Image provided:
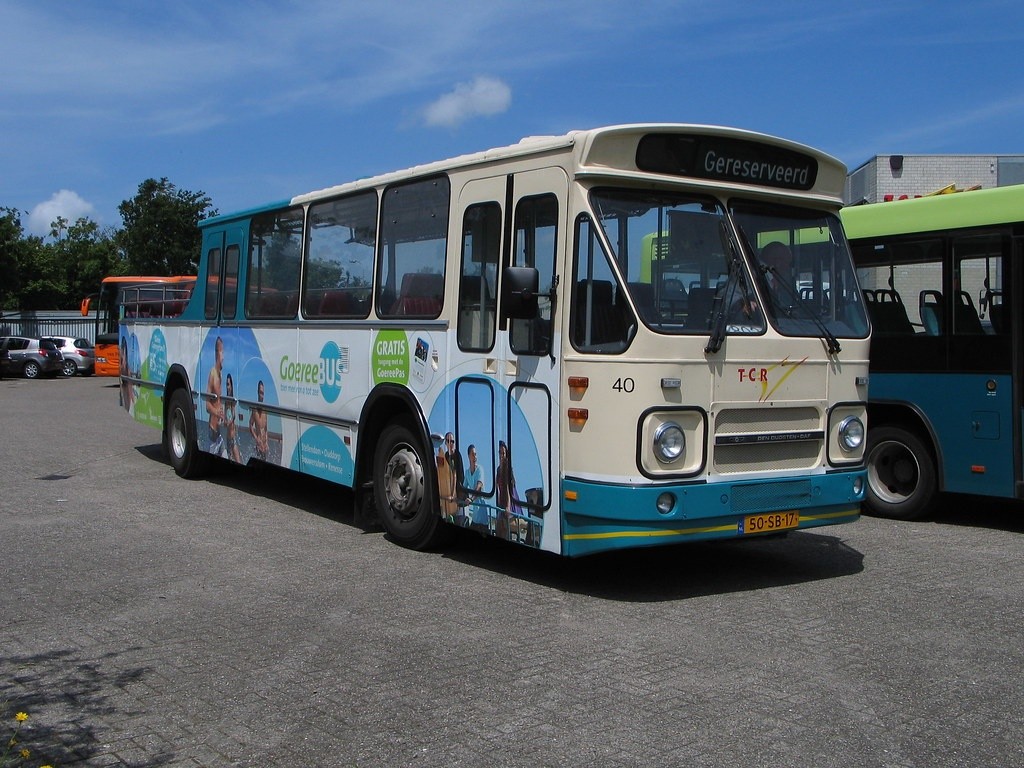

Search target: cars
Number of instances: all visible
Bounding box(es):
[0,334,94,379]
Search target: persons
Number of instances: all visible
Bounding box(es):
[205,337,269,461]
[714,241,791,323]
[442,432,523,541]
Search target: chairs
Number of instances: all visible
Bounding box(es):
[613,282,663,340]
[959,291,985,334]
[660,278,689,312]
[800,285,916,335]
[917,289,944,336]
[460,274,492,301]
[573,280,619,346]
[987,291,1004,335]
[389,271,444,317]
[317,291,354,315]
[205,294,317,316]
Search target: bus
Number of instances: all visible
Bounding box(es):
[115,118,876,565]
[640,184,1024,517]
[80,276,281,379]
